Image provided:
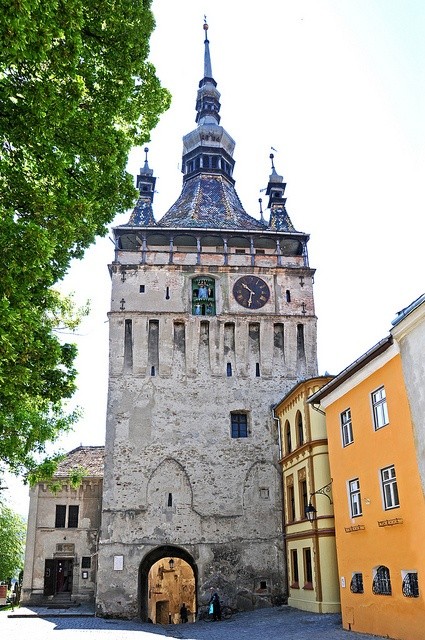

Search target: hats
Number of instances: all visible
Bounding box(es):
[214,593,217,596]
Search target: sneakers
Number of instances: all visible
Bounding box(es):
[211,619,215,622]
[218,619,222,621]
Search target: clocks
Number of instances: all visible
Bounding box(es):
[232,275,270,309]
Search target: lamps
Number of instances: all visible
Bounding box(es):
[306,478,333,523]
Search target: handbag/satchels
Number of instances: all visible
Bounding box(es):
[209,603,214,614]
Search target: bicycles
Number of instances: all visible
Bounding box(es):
[202,600,234,622]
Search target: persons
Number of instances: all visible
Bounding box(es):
[195,303,202,314]
[207,302,213,315]
[209,593,221,621]
[180,603,188,623]
